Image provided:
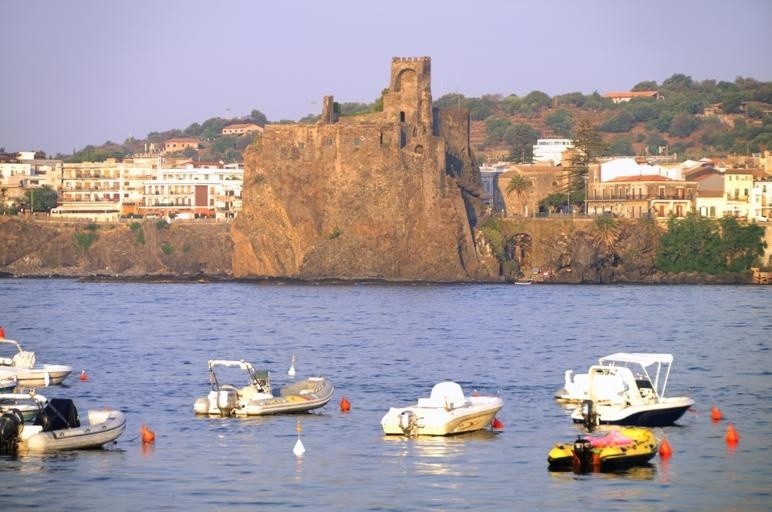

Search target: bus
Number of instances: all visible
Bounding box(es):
[45,204,120,223]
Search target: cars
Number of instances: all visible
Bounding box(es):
[602,210,616,217]
[95,272,112,279]
[566,267,573,272]
[140,212,162,219]
[754,214,768,221]
[531,267,541,275]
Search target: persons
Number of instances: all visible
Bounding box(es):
[537,200,585,216]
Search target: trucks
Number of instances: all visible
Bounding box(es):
[173,212,195,221]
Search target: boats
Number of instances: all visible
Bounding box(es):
[47,272,57,279]
[546,350,696,476]
[195,277,209,283]
[0,326,128,455]
[193,358,336,418]
[514,280,533,286]
[378,378,507,439]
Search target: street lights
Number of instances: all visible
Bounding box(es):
[162,196,169,218]
[567,190,575,214]
